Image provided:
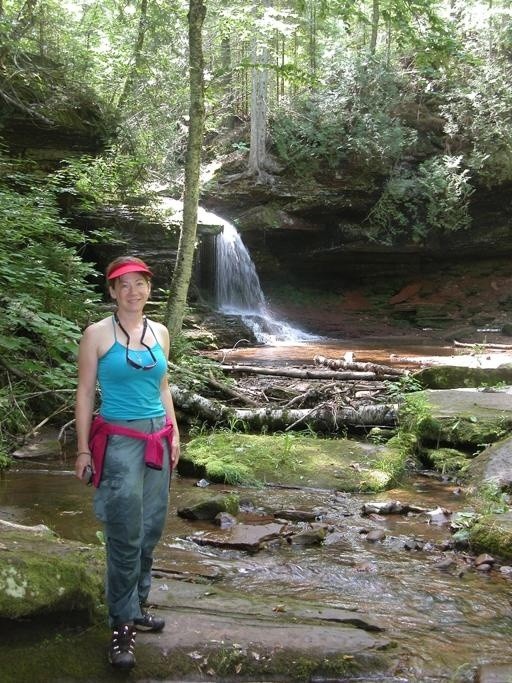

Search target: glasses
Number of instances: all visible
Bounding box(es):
[125,345,158,370]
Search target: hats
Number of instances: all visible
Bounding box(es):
[107,259,154,281]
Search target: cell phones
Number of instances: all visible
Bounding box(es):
[81,465,94,487]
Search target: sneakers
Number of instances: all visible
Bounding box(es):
[107,624,137,670]
[134,608,165,633]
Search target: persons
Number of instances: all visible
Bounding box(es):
[75,255,180,669]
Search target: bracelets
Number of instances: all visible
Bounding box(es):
[76,452,92,457]
